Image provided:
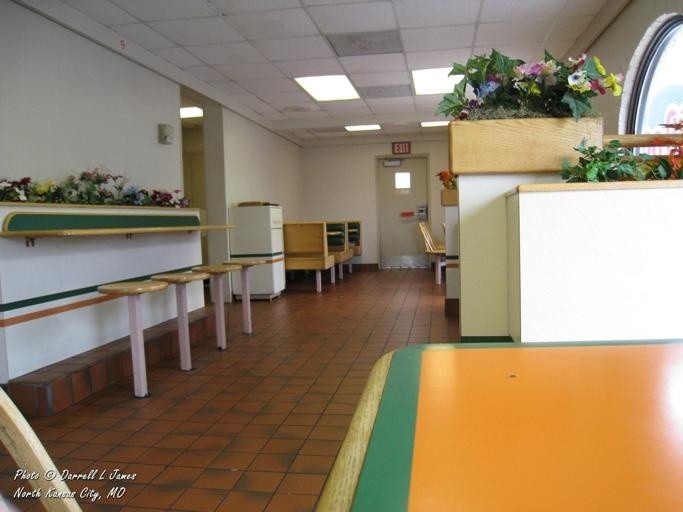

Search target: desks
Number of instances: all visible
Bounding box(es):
[311,339,682,510]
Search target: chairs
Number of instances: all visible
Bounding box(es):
[417,220,446,286]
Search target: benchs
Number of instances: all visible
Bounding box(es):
[283,219,364,292]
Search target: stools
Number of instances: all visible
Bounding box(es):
[98,260,266,399]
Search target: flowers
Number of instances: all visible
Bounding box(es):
[434,169,456,190]
[0,165,191,208]
[435,48,625,122]
